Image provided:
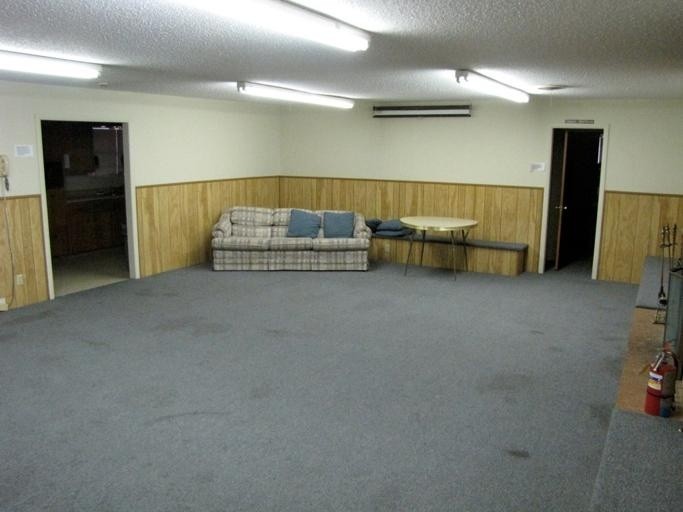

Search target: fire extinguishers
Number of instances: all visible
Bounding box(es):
[643,338,679,417]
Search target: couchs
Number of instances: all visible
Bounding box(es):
[211,206,373,270]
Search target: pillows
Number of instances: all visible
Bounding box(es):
[286,210,354,238]
[365,217,411,237]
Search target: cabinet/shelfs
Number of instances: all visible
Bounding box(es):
[67,199,125,256]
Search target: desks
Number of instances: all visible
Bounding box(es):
[398,216,477,281]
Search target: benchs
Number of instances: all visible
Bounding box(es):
[368,233,528,276]
